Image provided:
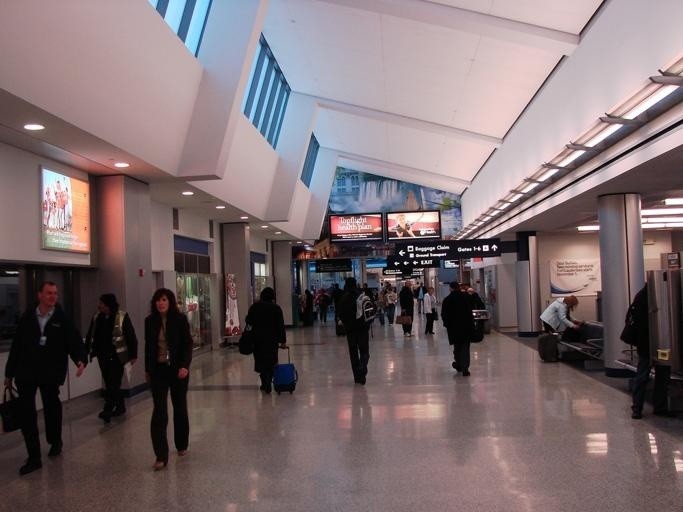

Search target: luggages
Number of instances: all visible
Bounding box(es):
[273,344,298,394]
[537,330,560,363]
[336,318,347,337]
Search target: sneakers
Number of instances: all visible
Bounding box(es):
[632,408,676,422]
[177,448,189,456]
[353,373,368,386]
[152,459,166,469]
[18,457,43,474]
[46,439,63,456]
[96,404,126,422]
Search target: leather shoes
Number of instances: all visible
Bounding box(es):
[451,361,471,376]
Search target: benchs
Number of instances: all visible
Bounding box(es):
[553,320,605,370]
[613,346,682,415]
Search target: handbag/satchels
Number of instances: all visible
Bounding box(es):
[0,381,25,433]
[237,321,257,356]
[395,311,412,325]
[620,302,639,348]
[470,321,484,344]
[432,307,439,321]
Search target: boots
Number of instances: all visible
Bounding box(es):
[260,377,272,394]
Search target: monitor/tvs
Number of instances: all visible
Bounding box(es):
[328,212,383,242]
[386,210,441,242]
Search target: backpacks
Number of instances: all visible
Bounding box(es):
[354,287,375,326]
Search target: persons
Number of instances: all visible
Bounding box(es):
[84,292,138,424]
[43,178,68,230]
[142,286,193,473]
[294,279,487,338]
[244,286,286,395]
[3,280,88,478]
[440,281,484,376]
[336,277,370,386]
[390,212,415,238]
[619,283,679,419]
[537,294,580,363]
